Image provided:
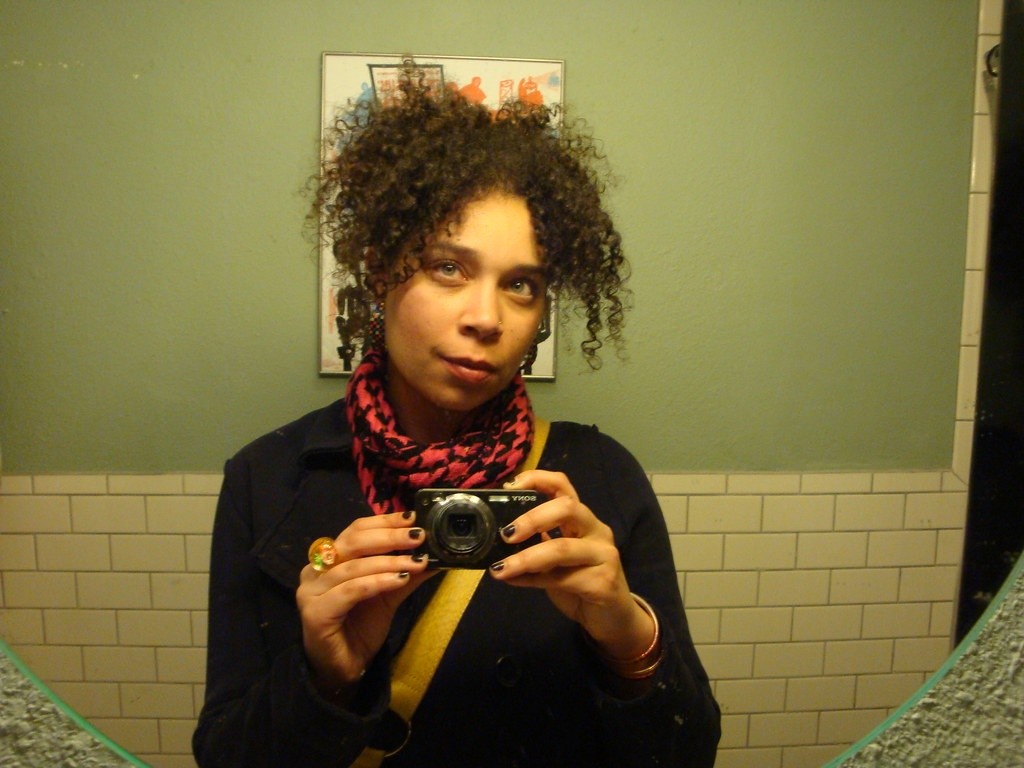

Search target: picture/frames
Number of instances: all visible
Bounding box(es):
[314,52,568,382]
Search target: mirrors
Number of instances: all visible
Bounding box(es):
[0,1,1023,767]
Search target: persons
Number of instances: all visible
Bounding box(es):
[192,76,723,768]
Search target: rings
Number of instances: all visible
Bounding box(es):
[308,538,339,571]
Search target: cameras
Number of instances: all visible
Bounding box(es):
[413,489,542,571]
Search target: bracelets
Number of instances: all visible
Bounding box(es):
[621,652,664,680]
[608,594,660,666]
